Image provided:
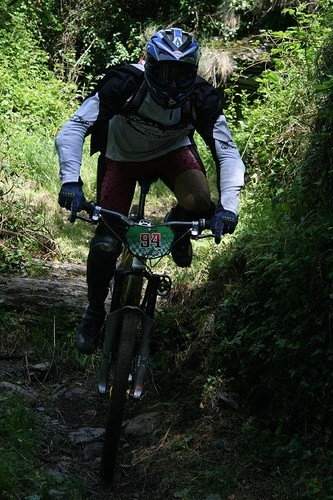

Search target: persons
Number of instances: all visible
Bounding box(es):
[54,28,245,353]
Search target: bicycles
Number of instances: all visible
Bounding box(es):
[64,176,215,487]
